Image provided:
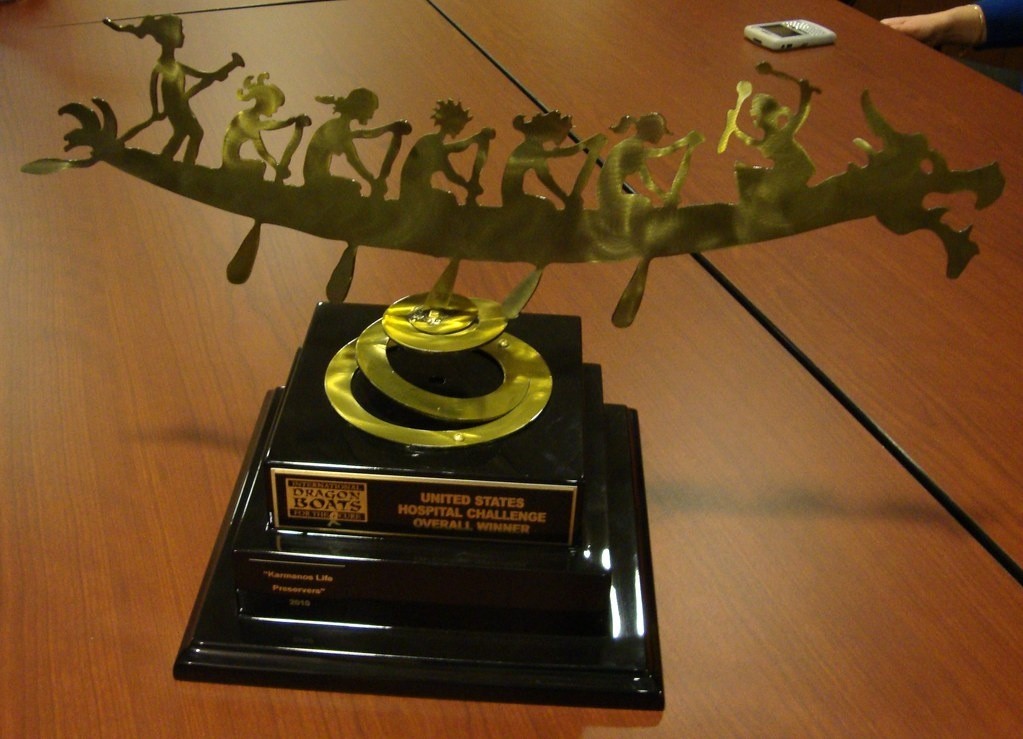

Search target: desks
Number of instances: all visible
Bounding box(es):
[1,1,1023,739]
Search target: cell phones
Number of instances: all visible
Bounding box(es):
[744,19,837,51]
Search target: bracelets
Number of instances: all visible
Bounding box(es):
[966,4,984,42]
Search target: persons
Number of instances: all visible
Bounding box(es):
[102,11,816,219]
[879,0,1023,52]
